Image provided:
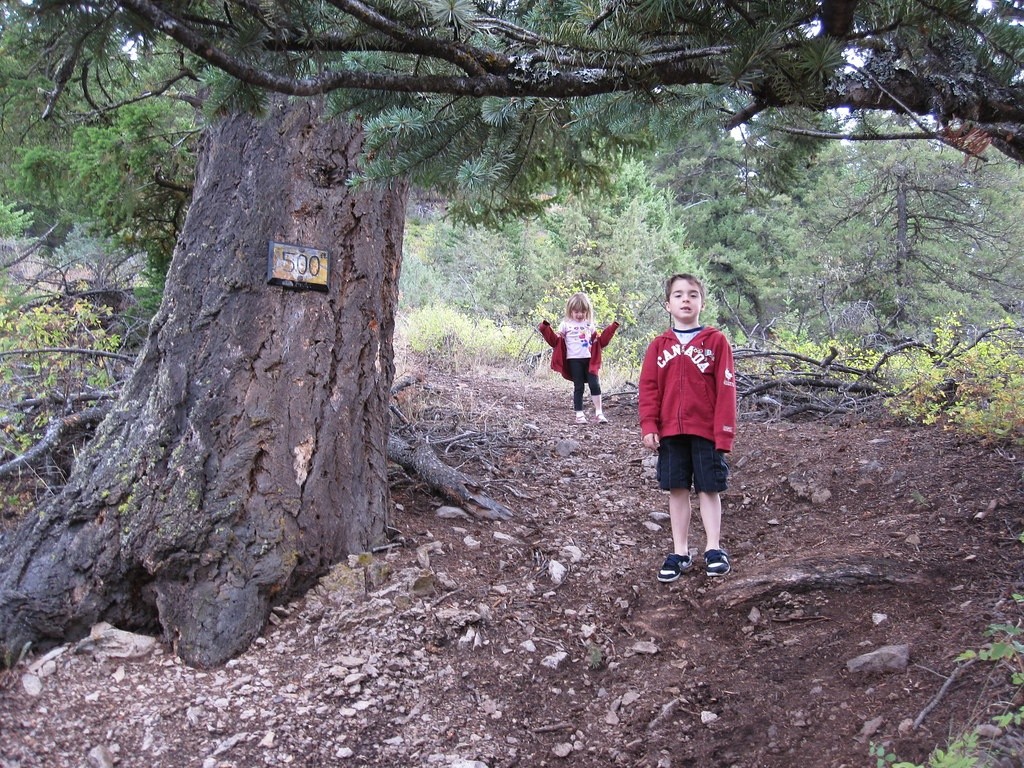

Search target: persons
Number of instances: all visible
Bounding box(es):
[537,292,620,424]
[638,274,736,582]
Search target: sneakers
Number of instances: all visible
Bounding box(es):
[704,548,731,577]
[576,417,587,423]
[657,550,693,583]
[596,414,608,423]
